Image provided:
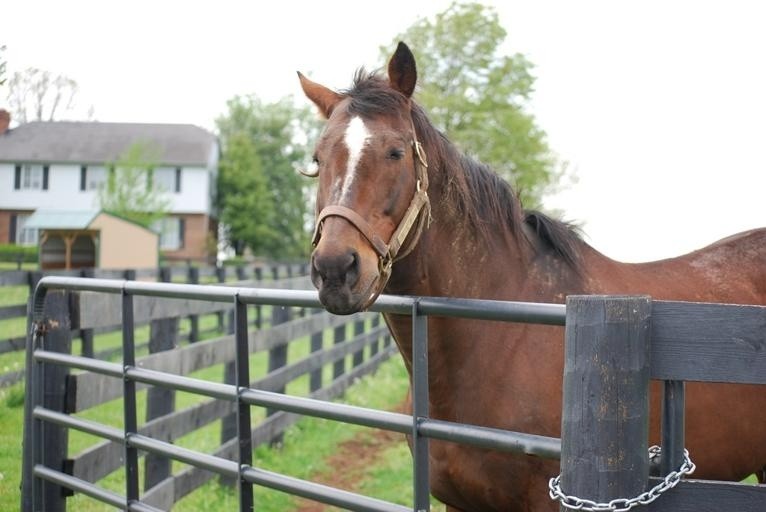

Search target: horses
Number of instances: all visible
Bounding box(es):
[296,40,766,512]
